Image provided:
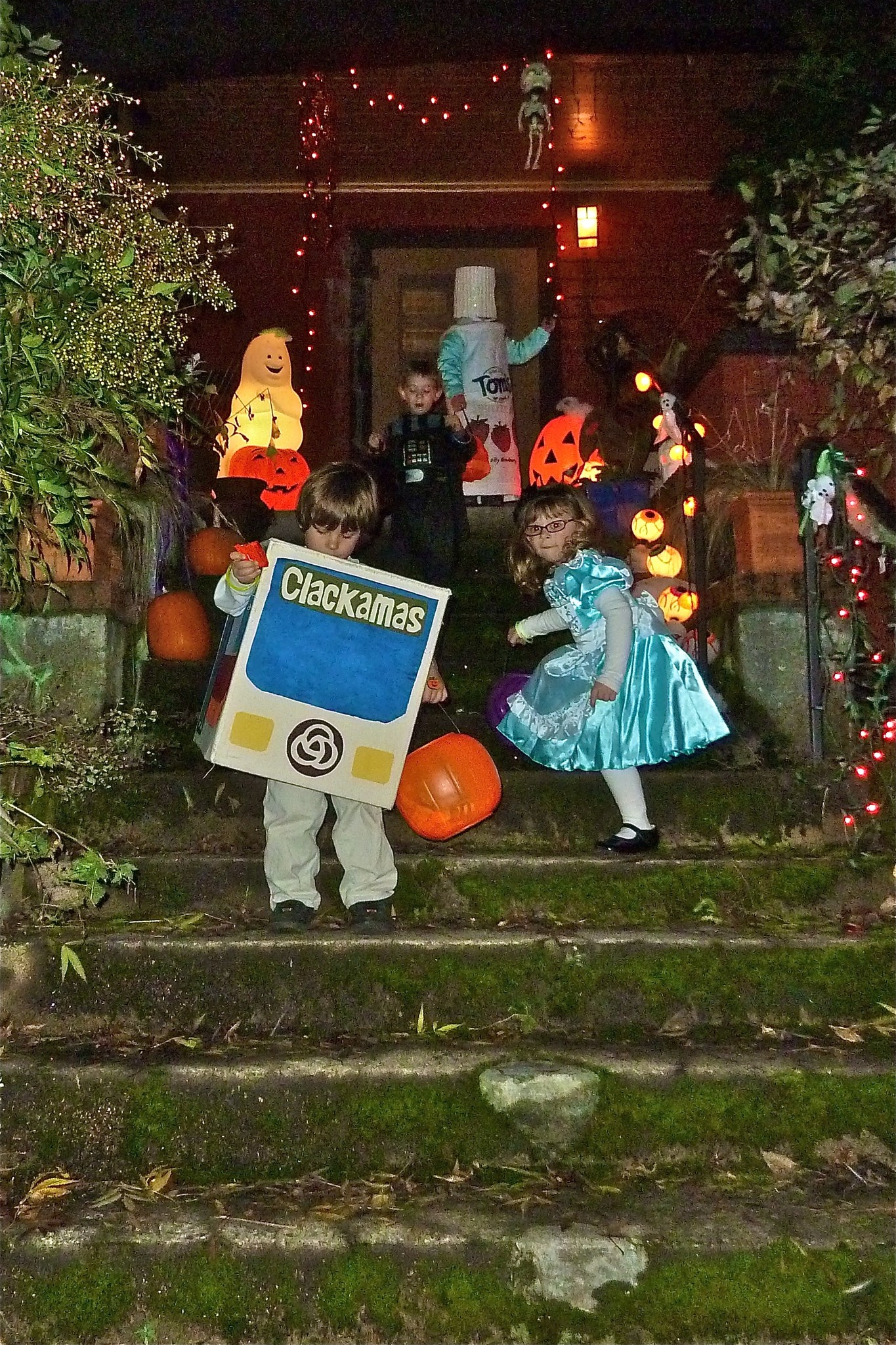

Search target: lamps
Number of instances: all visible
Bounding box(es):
[574,205,600,249]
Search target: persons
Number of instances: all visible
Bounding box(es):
[436,266,554,506]
[365,359,477,655]
[214,463,447,936]
[495,484,730,855]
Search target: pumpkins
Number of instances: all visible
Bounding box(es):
[147,585,211,660]
[189,506,245,576]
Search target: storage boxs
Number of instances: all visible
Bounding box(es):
[192,535,452,812]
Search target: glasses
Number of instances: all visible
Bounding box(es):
[523,517,574,537]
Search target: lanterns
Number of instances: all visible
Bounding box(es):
[228,446,311,511]
[529,415,604,488]
[397,732,503,841]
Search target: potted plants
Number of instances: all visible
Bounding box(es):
[683,363,822,574]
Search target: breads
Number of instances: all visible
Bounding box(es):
[235,541,269,567]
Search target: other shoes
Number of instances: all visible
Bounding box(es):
[594,822,660,853]
[348,899,393,935]
[268,900,317,931]
